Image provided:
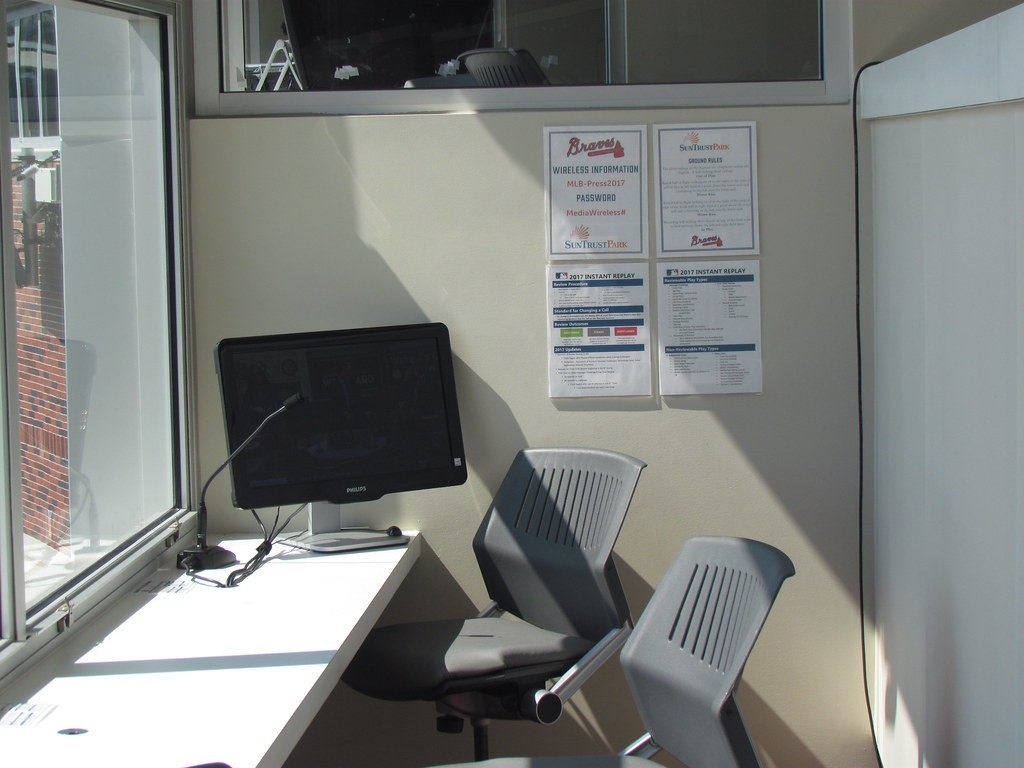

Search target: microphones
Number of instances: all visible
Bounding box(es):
[176,393,306,571]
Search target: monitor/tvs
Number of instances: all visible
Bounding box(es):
[213,322,468,553]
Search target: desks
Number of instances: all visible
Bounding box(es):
[0,531,421,768]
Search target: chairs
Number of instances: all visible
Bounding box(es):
[421,535,797,768]
[340,447,648,761]
